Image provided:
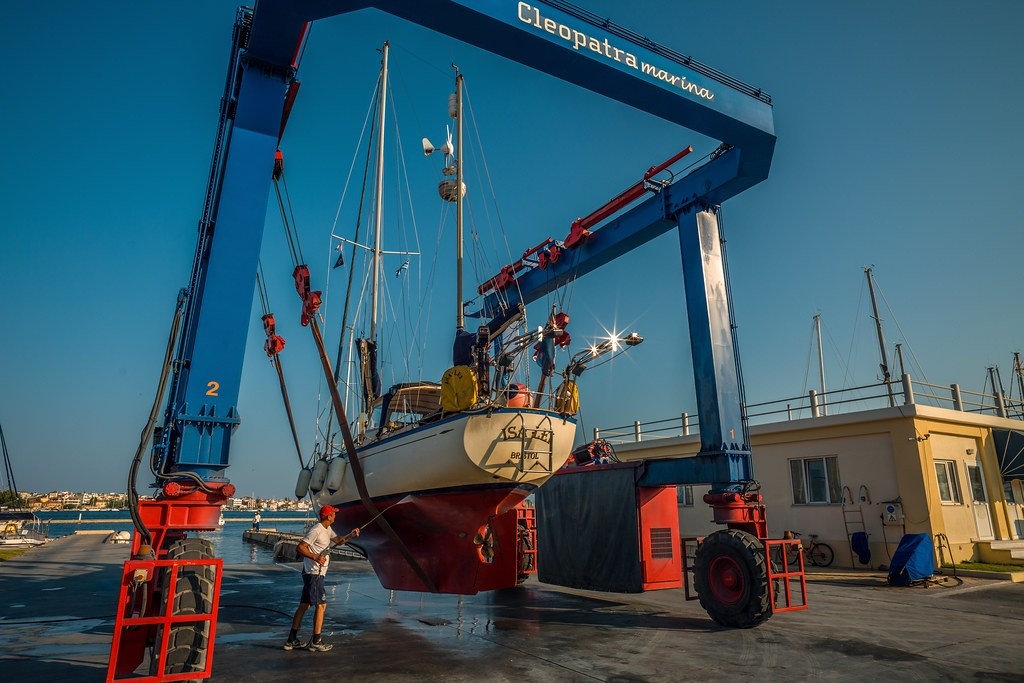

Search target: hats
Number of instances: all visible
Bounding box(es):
[319,506,339,518]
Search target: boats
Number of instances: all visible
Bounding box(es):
[0,528,48,550]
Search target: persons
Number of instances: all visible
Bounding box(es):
[256,511,261,532]
[283,506,360,651]
[253,513,257,533]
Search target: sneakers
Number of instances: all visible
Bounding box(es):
[308,637,333,652]
[283,639,307,650]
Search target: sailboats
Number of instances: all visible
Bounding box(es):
[290,42,647,507]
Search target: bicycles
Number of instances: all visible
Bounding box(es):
[778,531,834,567]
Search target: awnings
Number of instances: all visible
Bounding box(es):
[992,429,1024,482]
